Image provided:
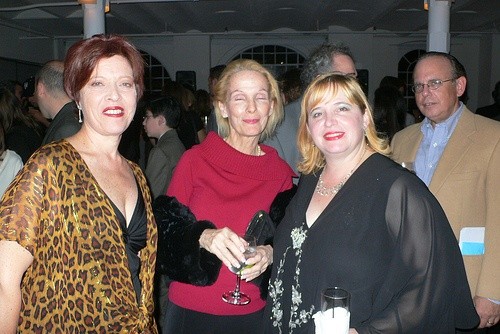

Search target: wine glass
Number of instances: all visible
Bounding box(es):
[221,234,257,305]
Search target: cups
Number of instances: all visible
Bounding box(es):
[320,287,351,318]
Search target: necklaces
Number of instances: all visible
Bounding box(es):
[316,145,368,196]
[223,136,261,156]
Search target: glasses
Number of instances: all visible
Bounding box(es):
[143,114,159,121]
[412,76,462,94]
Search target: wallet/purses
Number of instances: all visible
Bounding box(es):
[241,210,277,289]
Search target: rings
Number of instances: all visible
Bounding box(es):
[487,320,494,324]
[259,270,261,274]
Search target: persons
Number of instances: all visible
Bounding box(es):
[0,34,500,334]
